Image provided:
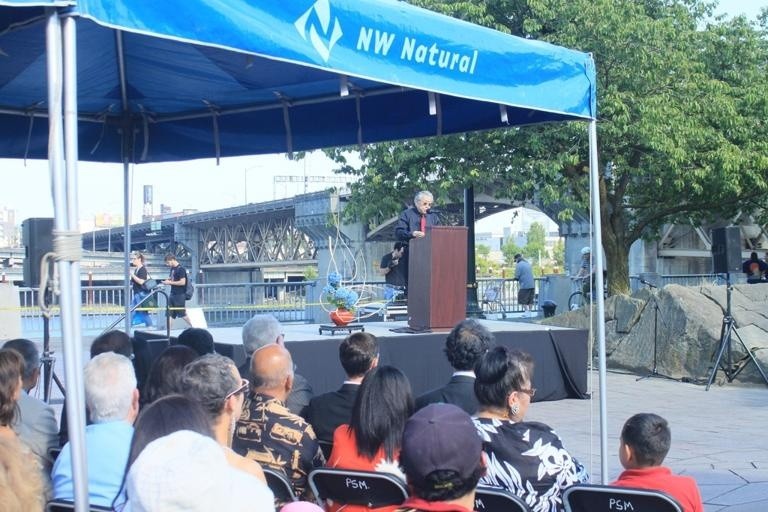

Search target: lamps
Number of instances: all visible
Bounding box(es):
[563,484,684,512]
[474,486,531,512]
[45,499,111,512]
[263,467,297,504]
[308,467,412,508]
[47,446,62,464]
[477,280,510,319]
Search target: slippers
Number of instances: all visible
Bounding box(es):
[176,266,194,300]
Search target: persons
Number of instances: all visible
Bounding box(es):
[394,403,487,512]
[0,349,42,512]
[742,251,767,283]
[412,318,493,414]
[160,254,193,328]
[395,191,442,238]
[130,253,157,327]
[308,332,380,458]
[379,241,409,300]
[174,325,216,358]
[606,412,704,512]
[51,351,141,510]
[326,365,413,512]
[182,354,271,488]
[468,345,589,512]
[574,247,597,295]
[513,254,535,318]
[227,343,326,500]
[57,331,137,450]
[237,313,314,415]
[2,338,57,471]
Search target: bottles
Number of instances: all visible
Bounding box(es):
[513,253,522,263]
[130,251,141,260]
[401,402,482,490]
[581,246,592,254]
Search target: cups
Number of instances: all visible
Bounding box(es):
[132,257,138,261]
[224,378,250,399]
[517,386,535,397]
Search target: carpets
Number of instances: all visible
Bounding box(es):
[420,214,427,232]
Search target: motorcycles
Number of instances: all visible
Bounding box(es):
[634,306,666,381]
[704,274,768,391]
[28,287,66,406]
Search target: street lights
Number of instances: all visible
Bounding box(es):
[143,268,158,291]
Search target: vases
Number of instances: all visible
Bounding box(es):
[323,271,359,310]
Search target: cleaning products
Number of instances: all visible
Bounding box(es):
[478,278,508,319]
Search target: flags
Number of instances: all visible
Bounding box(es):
[329,307,356,326]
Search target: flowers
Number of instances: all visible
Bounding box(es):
[640,279,656,288]
[426,209,441,214]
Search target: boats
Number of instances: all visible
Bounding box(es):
[712,226,743,274]
[21,217,55,287]
